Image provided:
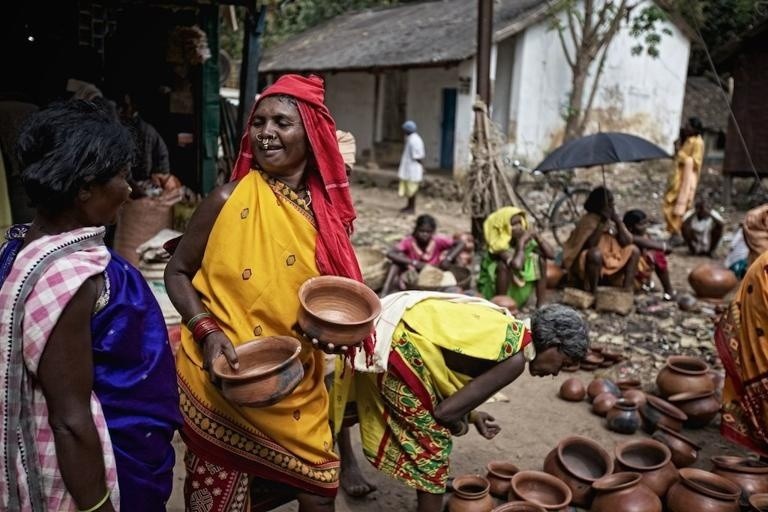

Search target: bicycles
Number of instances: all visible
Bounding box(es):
[501,156,594,247]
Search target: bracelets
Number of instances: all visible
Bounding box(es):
[187,313,222,342]
[411,260,418,267]
[86,488,113,512]
[462,412,471,422]
[663,241,667,251]
[446,256,453,262]
[540,240,545,245]
[455,421,468,437]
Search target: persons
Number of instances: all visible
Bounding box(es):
[120,104,170,181]
[336,128,376,496]
[381,214,467,298]
[353,290,591,511]
[720,248,768,457]
[164,76,367,509]
[681,192,726,259]
[665,116,706,243]
[398,121,427,213]
[486,208,555,311]
[0,100,183,512]
[624,210,679,304]
[563,187,639,293]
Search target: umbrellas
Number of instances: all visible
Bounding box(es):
[530,122,674,234]
